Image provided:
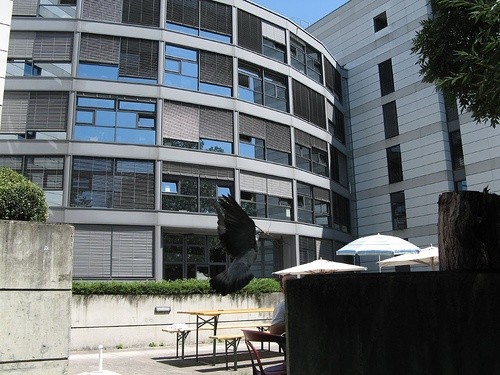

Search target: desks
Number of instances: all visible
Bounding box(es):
[177,309,274,366]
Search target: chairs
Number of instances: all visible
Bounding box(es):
[243,329,289,375]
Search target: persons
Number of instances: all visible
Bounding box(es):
[270,274,297,335]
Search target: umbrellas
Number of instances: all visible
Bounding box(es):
[376,244,440,271]
[336,233,421,272]
[273,257,367,279]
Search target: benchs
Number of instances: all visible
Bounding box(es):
[210,333,242,371]
[163,322,271,360]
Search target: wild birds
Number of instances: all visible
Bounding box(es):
[209,193,289,296]
